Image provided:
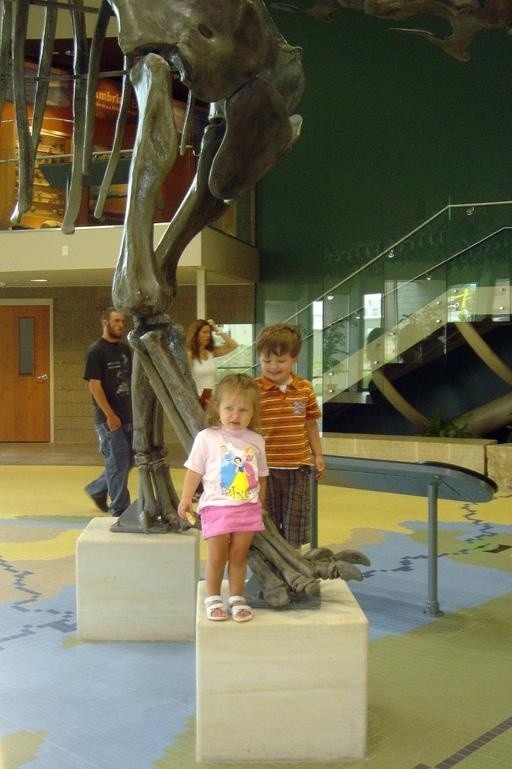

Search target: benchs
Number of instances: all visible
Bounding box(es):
[39,158,133,226]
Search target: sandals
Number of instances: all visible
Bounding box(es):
[226,595,253,621]
[205,596,229,621]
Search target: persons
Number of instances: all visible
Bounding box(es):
[184,317,238,398]
[249,318,325,547]
[78,305,135,518]
[177,372,271,623]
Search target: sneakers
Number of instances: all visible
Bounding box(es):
[85,487,108,512]
